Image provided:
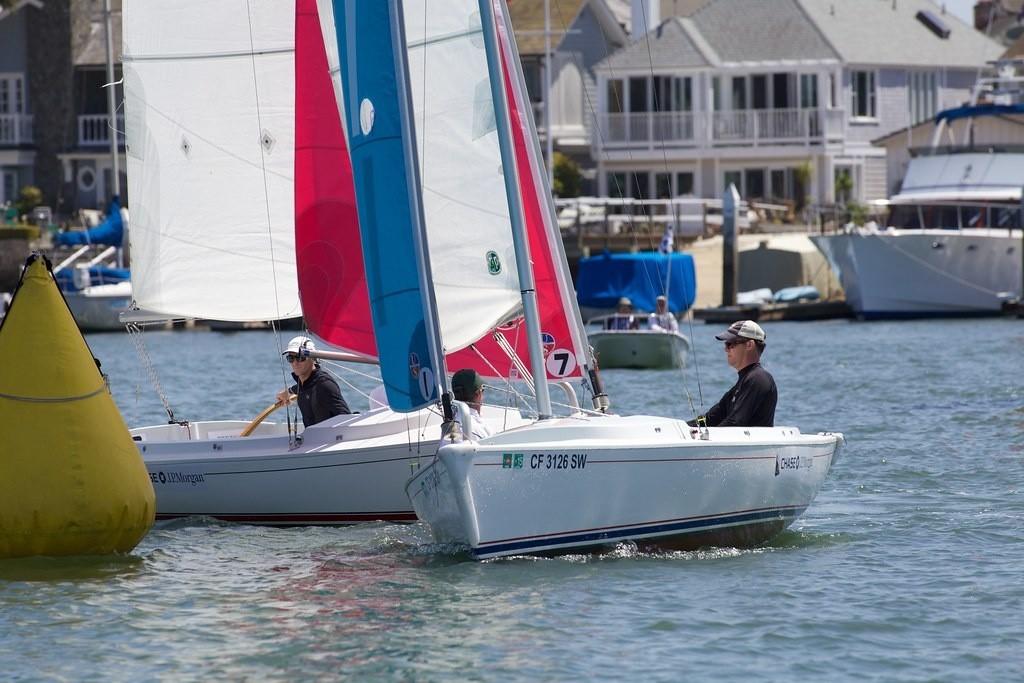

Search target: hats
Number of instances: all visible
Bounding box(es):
[281,336,315,355]
[618,297,632,306]
[657,295,666,301]
[451,369,488,396]
[715,320,767,342]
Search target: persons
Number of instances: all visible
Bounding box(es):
[603,297,641,330]
[451,369,490,440]
[686,319,778,426]
[276,336,352,428]
[648,295,679,331]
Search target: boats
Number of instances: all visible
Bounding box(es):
[809,60,1024,316]
[586,313,689,368]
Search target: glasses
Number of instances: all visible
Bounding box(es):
[725,340,746,349]
[285,355,306,363]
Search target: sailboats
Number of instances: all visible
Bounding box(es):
[119,2,550,519]
[337,2,843,561]
[19,0,205,331]
